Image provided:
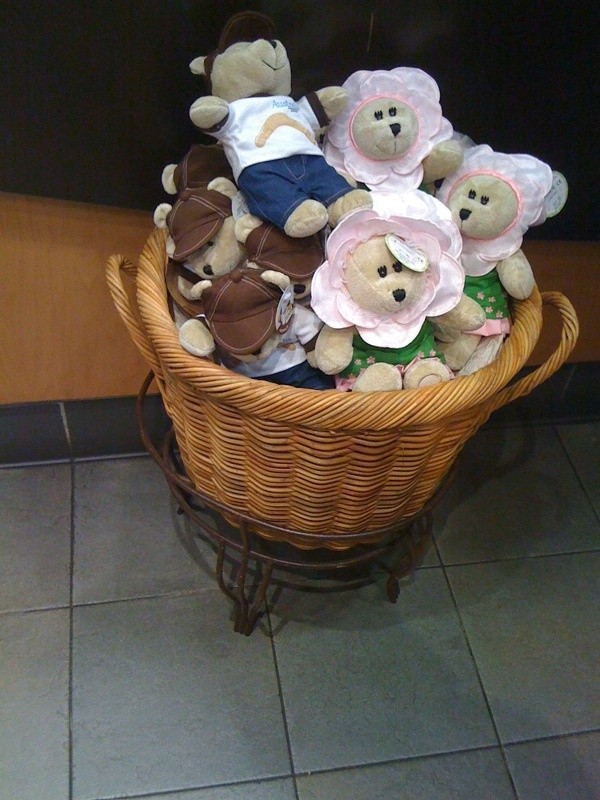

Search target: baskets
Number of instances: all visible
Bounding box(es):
[105,222,581,552]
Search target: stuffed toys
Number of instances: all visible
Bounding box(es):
[153,31,568,391]
